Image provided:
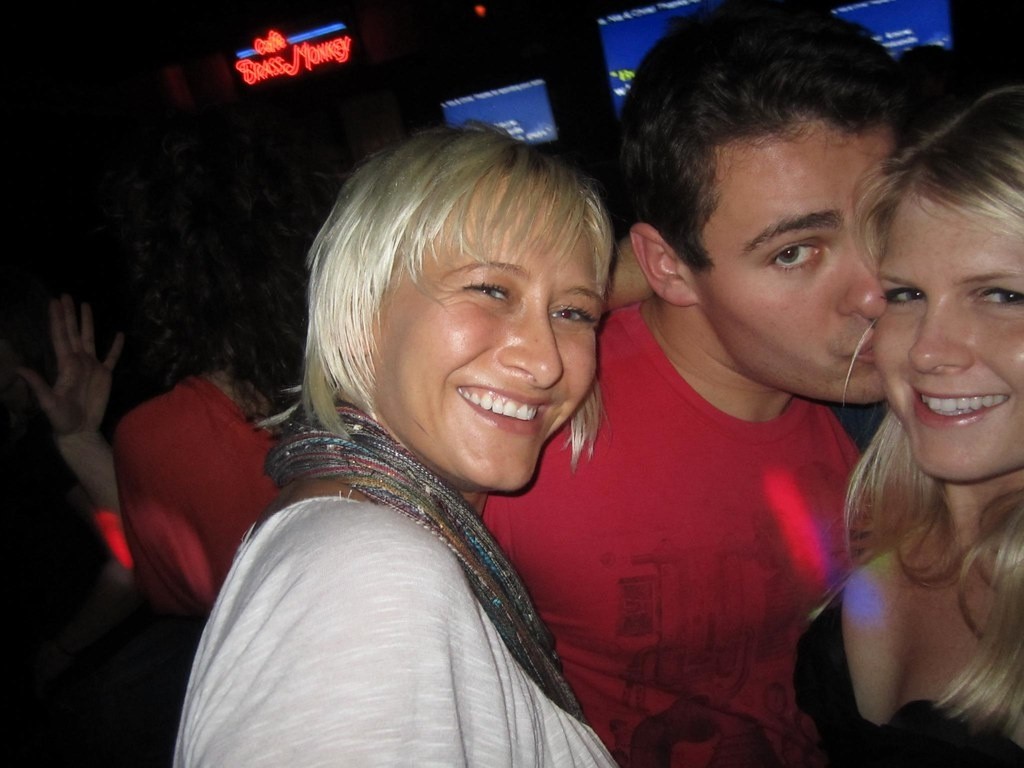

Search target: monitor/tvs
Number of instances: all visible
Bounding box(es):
[830,0,954,61]
[598,0,722,119]
[441,78,558,146]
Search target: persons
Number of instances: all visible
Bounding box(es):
[479,1,904,768]
[18,115,340,688]
[793,85,1024,768]
[173,120,618,768]
